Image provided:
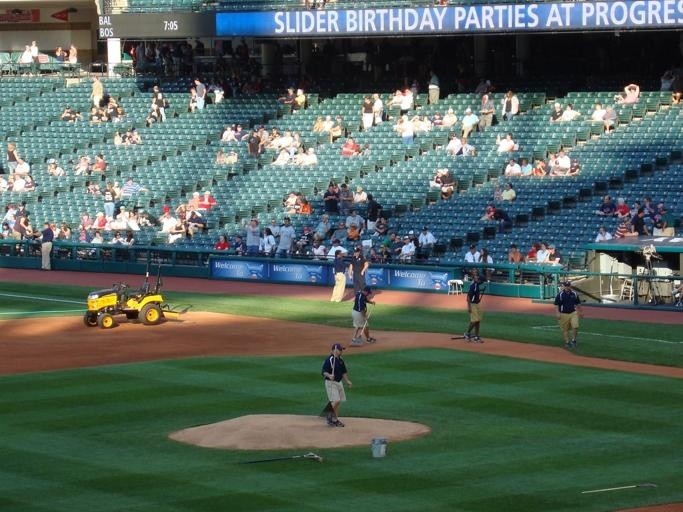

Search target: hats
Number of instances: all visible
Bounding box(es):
[331,344,346,351]
[468,240,562,256]
[363,285,371,294]
[244,207,430,253]
[563,281,571,286]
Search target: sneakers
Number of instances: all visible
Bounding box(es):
[474,336,484,343]
[352,337,377,344]
[327,416,344,428]
[463,331,470,341]
[564,341,570,349]
[571,338,578,347]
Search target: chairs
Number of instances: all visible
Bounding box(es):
[0,54,681,293]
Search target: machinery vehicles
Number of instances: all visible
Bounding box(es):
[83,255,172,328]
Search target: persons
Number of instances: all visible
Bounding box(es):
[321,343,354,428]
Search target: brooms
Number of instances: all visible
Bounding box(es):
[351,304,373,346]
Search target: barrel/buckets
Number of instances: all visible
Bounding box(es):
[371,437,388,457]
